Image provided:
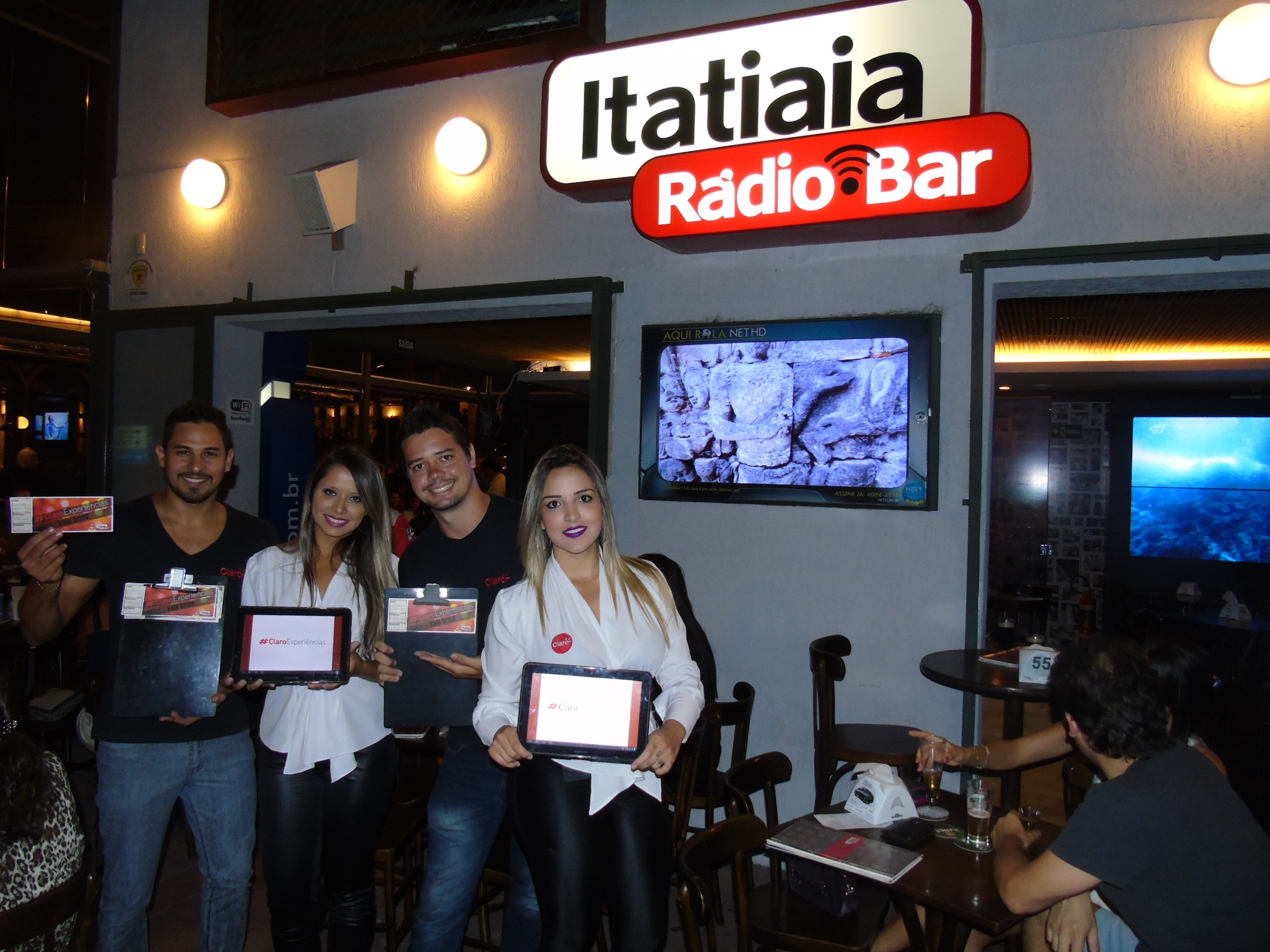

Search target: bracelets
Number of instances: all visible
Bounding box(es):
[37,578,61,593]
[975,744,991,769]
[967,745,980,765]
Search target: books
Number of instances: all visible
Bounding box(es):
[767,819,926,884]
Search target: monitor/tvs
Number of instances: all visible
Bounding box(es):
[31,410,74,442]
[639,313,939,510]
[1109,406,1270,578]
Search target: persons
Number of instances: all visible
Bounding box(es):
[17,399,707,952]
[864,626,1269,952]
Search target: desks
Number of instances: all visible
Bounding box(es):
[768,789,1062,952]
[920,650,1052,811]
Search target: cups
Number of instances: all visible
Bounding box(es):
[996,610,1018,650]
[966,779,993,845]
[1025,634,1045,646]
[1017,806,1042,831]
[918,733,946,812]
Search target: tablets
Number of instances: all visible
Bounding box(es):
[229,606,351,684]
[516,661,653,765]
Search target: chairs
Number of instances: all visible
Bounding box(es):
[378,634,943,952]
